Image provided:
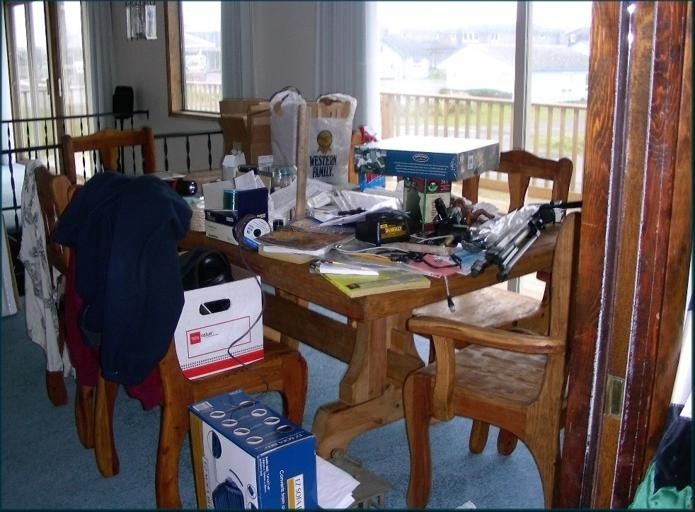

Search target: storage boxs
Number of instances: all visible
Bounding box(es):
[202,174,272,246]
[185,390,318,512]
[173,250,264,382]
[218,100,273,165]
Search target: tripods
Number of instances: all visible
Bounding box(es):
[470,203,556,283]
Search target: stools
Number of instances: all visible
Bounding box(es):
[318,456,394,510]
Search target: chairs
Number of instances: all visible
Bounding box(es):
[347,129,361,185]
[50,176,308,512]
[31,164,96,450]
[60,125,155,187]
[427,150,573,455]
[402,211,582,510]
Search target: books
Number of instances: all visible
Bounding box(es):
[255,216,432,300]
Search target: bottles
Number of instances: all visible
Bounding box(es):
[164,179,197,195]
[272,165,297,224]
[237,165,258,179]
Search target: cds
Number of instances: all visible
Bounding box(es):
[243,218,271,250]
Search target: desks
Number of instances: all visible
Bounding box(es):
[179,172,562,460]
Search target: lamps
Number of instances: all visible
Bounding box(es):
[125,0,158,41]
